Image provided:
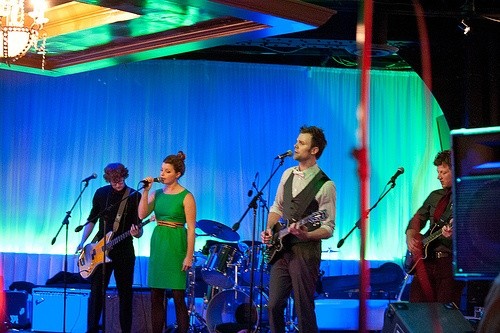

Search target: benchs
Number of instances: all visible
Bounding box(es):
[0,252,407,333]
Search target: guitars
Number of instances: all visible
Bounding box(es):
[262,208,329,265]
[402,221,454,275]
[77,215,155,280]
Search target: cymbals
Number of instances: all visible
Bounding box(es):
[195,219,240,241]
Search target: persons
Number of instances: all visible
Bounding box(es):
[76,163,143,333]
[405,150,466,307]
[138,151,197,333]
[261,124,337,333]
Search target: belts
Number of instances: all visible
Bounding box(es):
[428,252,450,258]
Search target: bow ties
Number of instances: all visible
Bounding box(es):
[292,169,304,179]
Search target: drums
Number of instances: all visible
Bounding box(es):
[201,244,246,289]
[205,286,270,333]
[242,243,271,286]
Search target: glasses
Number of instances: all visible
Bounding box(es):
[112,180,123,185]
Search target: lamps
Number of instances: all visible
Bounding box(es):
[0,0,49,73]
[458,19,470,35]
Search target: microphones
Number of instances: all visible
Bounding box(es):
[248,172,258,197]
[82,173,97,182]
[275,150,293,159]
[387,167,404,185]
[141,177,162,183]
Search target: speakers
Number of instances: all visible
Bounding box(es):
[104,285,168,333]
[450,124,500,280]
[32,284,92,333]
[381,301,476,333]
